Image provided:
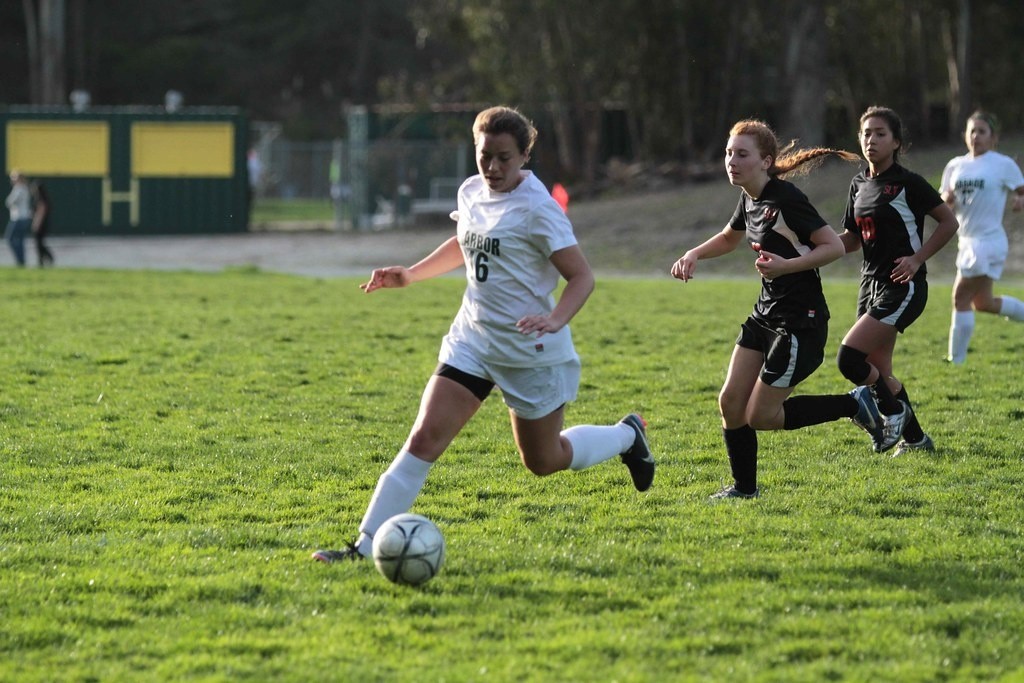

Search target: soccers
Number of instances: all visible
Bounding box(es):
[372,513,446,586]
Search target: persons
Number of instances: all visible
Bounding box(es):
[938,111,1024,365]
[6,174,54,265]
[313,106,655,562]
[838,108,959,456]
[671,121,884,501]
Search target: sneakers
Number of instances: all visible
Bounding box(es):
[876,399,911,452]
[848,384,886,451]
[892,434,935,459]
[313,535,364,564]
[618,413,654,491]
[709,477,760,503]
